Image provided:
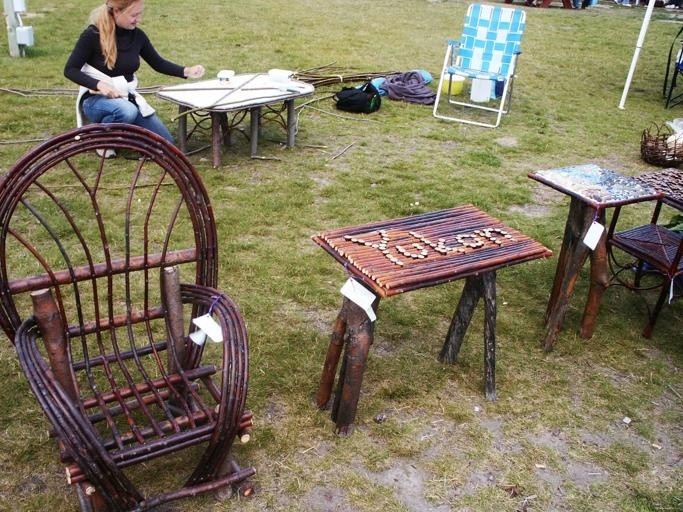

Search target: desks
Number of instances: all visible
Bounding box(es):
[153,72,316,169]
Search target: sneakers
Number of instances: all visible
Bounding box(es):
[96,149,117,158]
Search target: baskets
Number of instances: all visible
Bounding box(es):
[640,122,682,167]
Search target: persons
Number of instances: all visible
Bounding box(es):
[61,1,206,162]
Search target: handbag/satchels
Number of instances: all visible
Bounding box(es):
[332,81,381,114]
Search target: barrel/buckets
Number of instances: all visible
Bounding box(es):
[469,78,492,103]
[441,73,465,96]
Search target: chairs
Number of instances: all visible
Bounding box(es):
[432,2,527,129]
[1,122,256,511]
